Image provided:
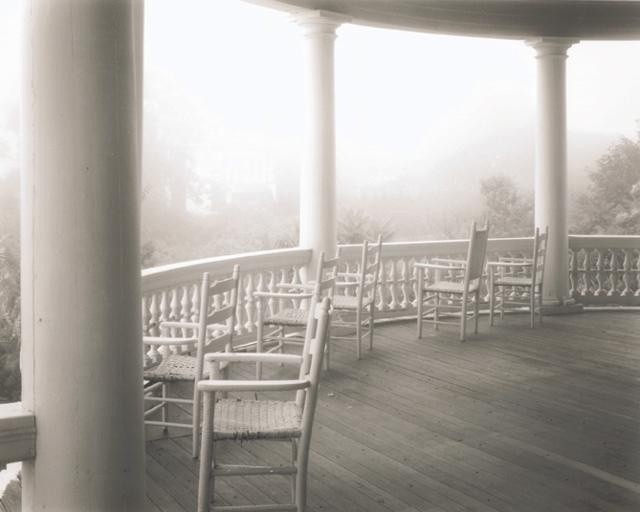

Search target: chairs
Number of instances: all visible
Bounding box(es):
[324,236,382,360]
[197,297,331,512]
[143,265,240,461]
[255,245,344,382]
[415,222,550,343]
